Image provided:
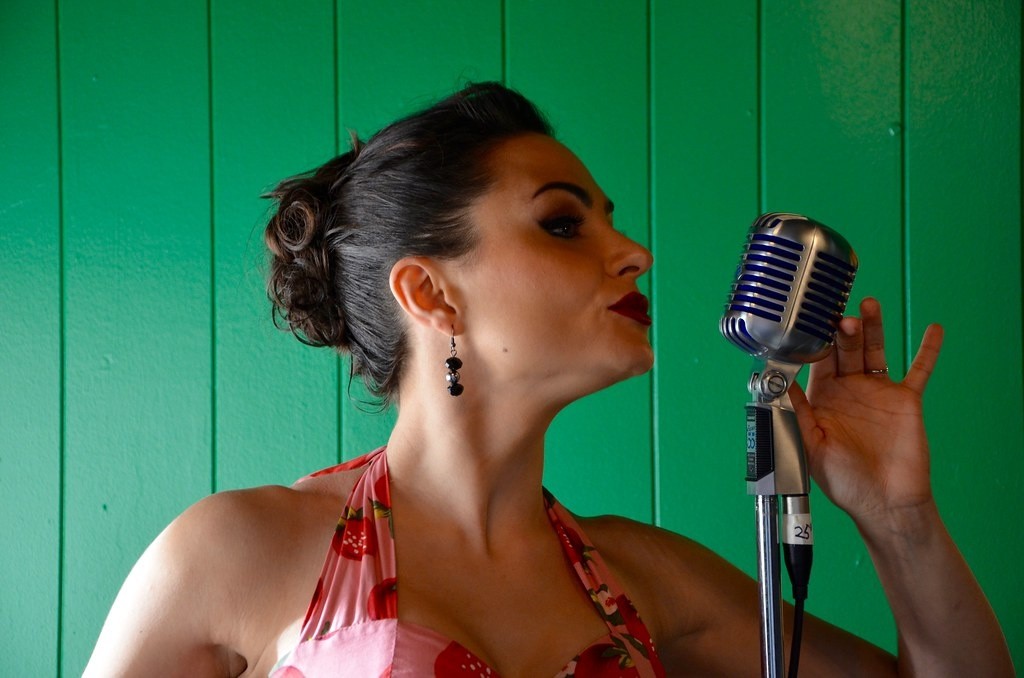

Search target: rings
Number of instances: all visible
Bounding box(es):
[865,365,889,374]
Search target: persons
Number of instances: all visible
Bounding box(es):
[79,82,1016,678]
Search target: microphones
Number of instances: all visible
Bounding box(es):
[720,212,858,601]
